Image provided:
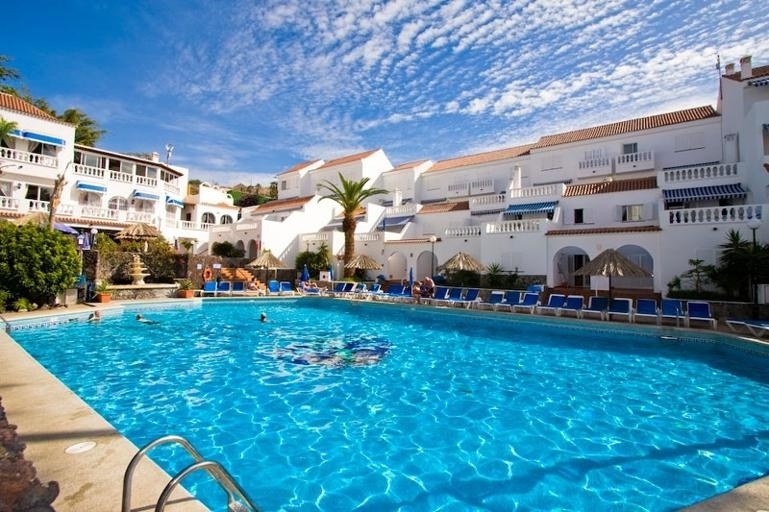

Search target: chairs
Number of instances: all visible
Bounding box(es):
[538,294,718,331]
[365,283,538,315]
[201,278,364,298]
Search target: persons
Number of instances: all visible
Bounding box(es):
[311,282,329,290]
[413,280,423,304]
[303,282,320,292]
[424,276,436,298]
[260,312,266,321]
[135,313,159,326]
[403,279,411,296]
[86,310,101,323]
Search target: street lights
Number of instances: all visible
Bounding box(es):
[747,214,760,322]
[428,234,437,282]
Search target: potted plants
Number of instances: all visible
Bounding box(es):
[91,279,116,303]
[176,279,194,298]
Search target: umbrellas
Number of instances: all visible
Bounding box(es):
[570,248,655,321]
[343,254,384,282]
[244,251,289,296]
[436,251,489,307]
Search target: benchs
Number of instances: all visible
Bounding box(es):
[726,317,768,339]
